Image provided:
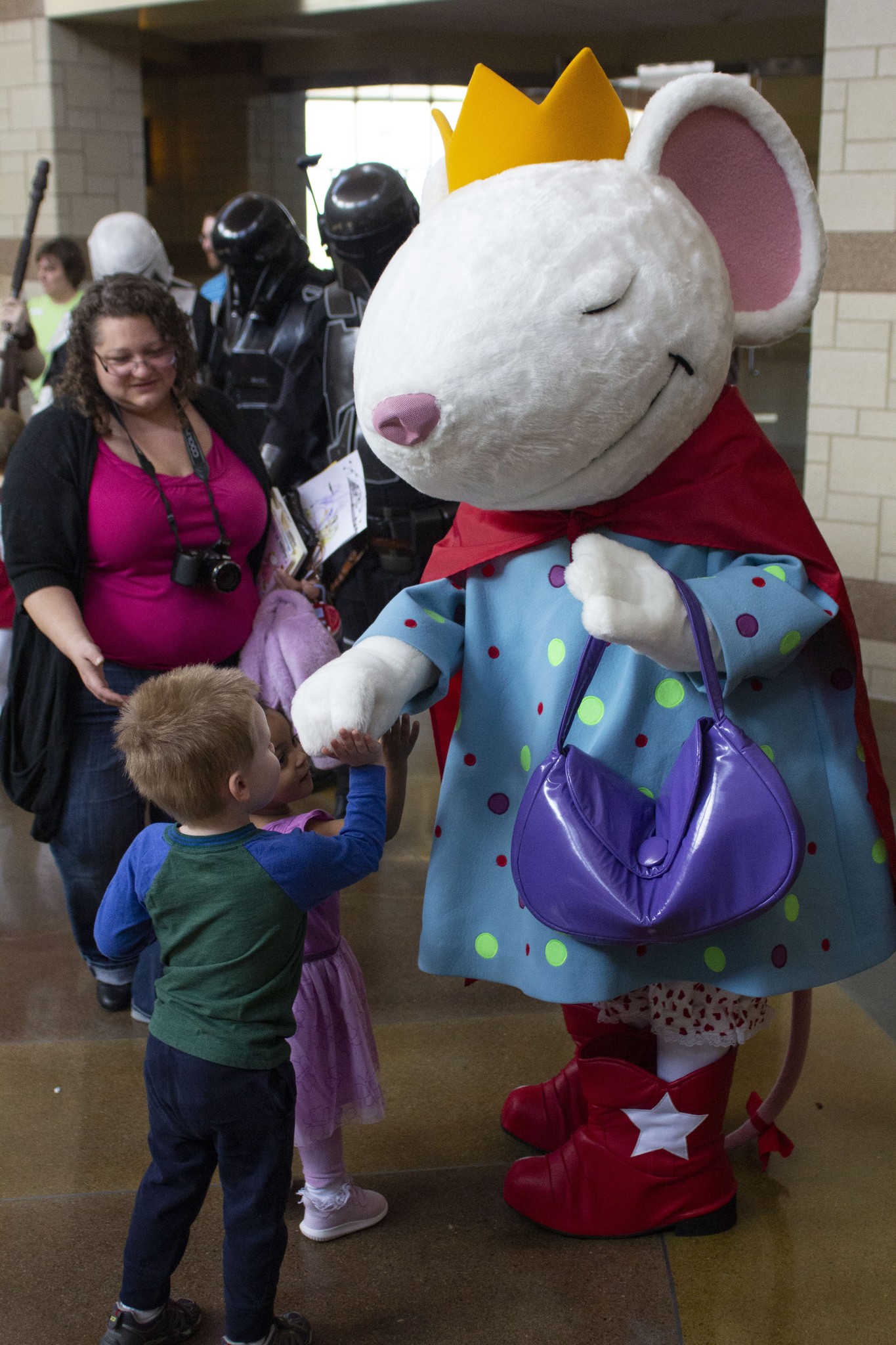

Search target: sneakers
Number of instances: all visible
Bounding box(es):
[296,1182,387,1241]
[221,1311,312,1345]
[100,1298,203,1345]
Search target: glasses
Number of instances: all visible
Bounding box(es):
[91,348,176,376]
[198,234,213,244]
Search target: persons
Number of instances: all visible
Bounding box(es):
[94,664,388,1345]
[258,163,461,659]
[0,297,46,380]
[249,703,418,1240]
[204,193,328,443]
[200,204,227,300]
[31,212,220,417]
[0,272,271,1023]
[23,236,86,403]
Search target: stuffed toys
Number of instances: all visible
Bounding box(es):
[291,47,896,1239]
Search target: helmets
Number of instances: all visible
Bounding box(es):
[213,192,310,325]
[87,212,173,291]
[317,163,420,300]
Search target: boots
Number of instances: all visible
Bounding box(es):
[502,999,651,1153]
[503,1031,739,1238]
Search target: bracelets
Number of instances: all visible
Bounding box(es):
[17,327,35,349]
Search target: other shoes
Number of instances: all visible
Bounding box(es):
[334,785,350,821]
[308,765,337,793]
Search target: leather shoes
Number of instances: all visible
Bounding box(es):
[96,980,132,1010]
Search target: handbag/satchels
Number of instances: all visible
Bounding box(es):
[511,567,803,946]
